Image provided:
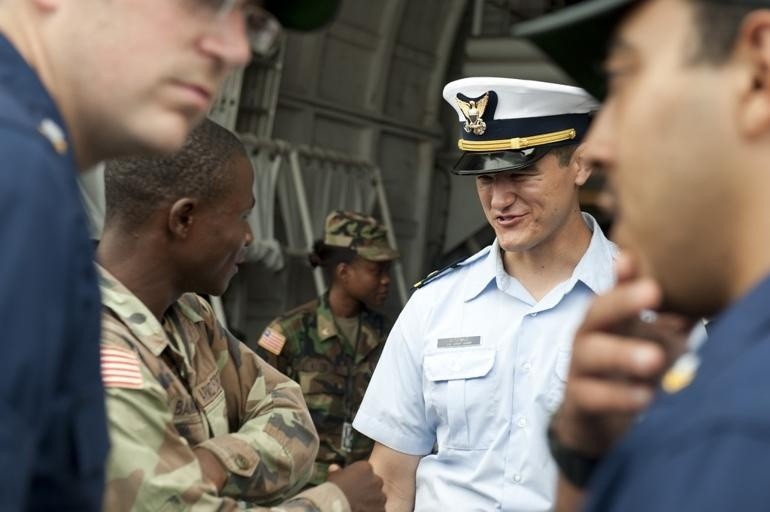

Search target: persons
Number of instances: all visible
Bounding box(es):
[348,74,709,512]
[542,2,769,512]
[253,207,402,494]
[0,0,342,511]
[91,109,388,511]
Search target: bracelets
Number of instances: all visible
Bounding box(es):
[546,409,610,491]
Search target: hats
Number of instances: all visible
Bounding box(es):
[512,1,644,104]
[442,77,600,175]
[252,0,342,34]
[324,209,401,261]
